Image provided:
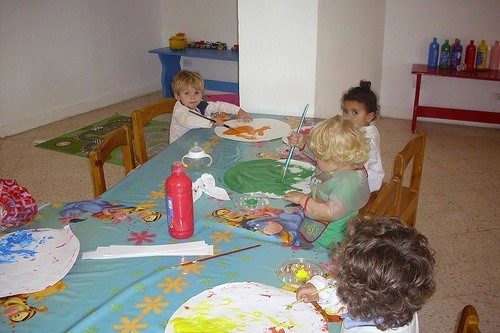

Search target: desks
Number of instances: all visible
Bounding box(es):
[0,113,341,333]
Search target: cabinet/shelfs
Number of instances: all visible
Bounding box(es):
[148,47,239,99]
[411,64,500,134]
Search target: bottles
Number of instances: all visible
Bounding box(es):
[189,40,239,52]
[475,39,488,69]
[450,38,463,70]
[489,40,500,70]
[181,141,213,170]
[464,40,477,70]
[427,37,439,67]
[438,39,450,68]
[165,161,193,240]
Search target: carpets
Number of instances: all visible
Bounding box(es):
[33,113,171,166]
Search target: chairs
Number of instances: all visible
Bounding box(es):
[131,98,178,164]
[89,124,135,197]
[361,132,426,230]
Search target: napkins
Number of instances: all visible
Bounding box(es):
[183,174,235,204]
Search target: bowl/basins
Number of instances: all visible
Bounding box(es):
[168,35,187,50]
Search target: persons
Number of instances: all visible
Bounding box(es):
[169,70,253,145]
[0,178,38,228]
[340,80,385,193]
[282,114,370,249]
[295,212,438,333]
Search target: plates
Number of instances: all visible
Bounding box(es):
[274,144,301,158]
[276,258,324,289]
[235,193,270,213]
